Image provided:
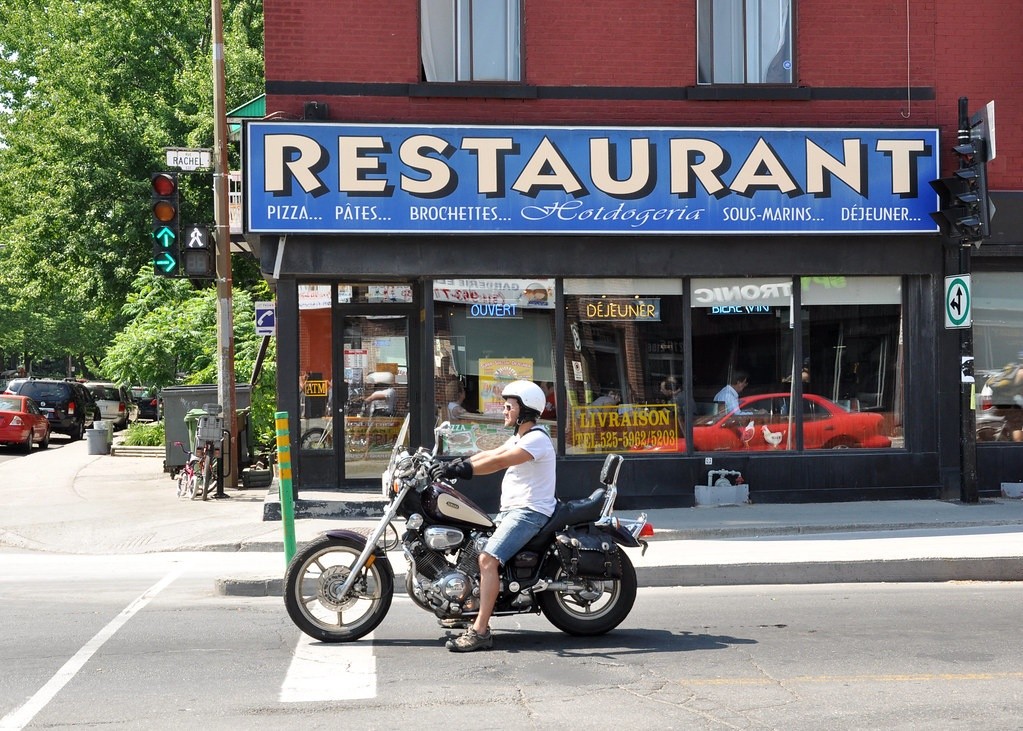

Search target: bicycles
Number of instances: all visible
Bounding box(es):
[198,438,219,502]
[173,441,205,499]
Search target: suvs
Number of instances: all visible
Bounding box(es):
[18,376,102,440]
[3,378,29,397]
[974,369,1023,432]
[130,385,163,421]
[83,380,142,430]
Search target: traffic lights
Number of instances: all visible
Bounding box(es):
[149,170,179,277]
[951,138,993,239]
[184,223,212,276]
[929,175,964,242]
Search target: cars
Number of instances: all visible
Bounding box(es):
[628,393,894,455]
[0,394,52,453]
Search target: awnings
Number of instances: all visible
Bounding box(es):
[225,94,266,141]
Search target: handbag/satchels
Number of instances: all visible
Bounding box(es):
[556,534,622,580]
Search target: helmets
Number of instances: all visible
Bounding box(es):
[369,371,395,384]
[501,379,547,415]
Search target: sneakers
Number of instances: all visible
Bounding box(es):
[437,617,476,629]
[445,624,494,651]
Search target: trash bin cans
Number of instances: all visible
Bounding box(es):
[85,429,108,455]
[161,384,254,479]
[184,408,210,456]
[92,420,114,445]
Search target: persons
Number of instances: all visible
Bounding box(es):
[446,381,469,420]
[586,384,622,413]
[660,376,698,417]
[361,372,397,460]
[539,382,557,420]
[713,368,768,416]
[429,380,556,652]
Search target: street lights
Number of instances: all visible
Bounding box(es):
[832,344,847,403]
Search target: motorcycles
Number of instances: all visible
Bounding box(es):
[282,413,655,641]
[298,389,402,453]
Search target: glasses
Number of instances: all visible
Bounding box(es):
[502,404,513,413]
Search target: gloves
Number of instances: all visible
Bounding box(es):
[426,457,473,482]
